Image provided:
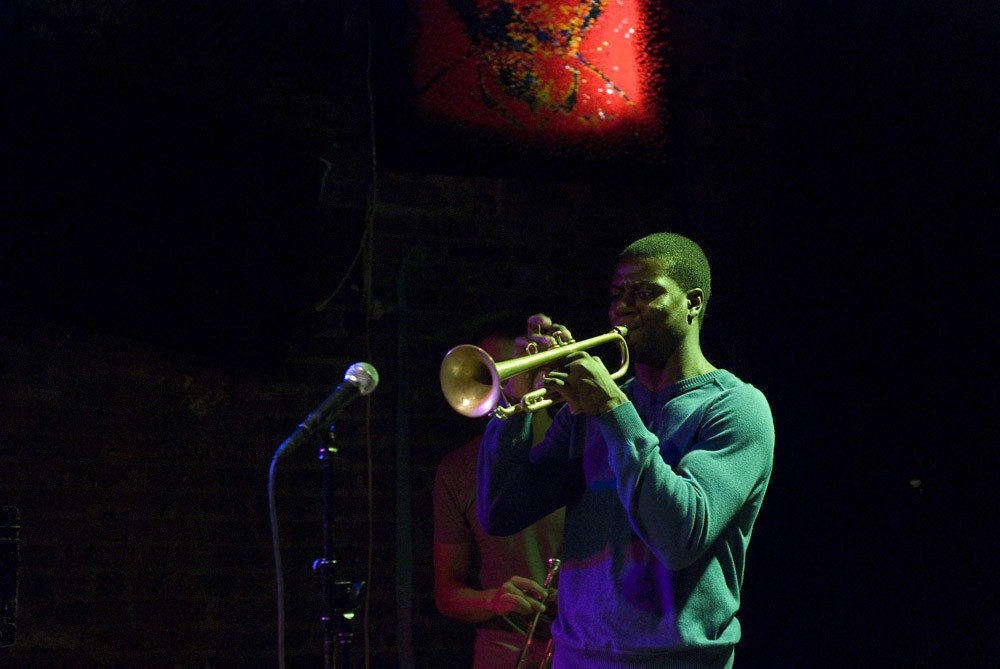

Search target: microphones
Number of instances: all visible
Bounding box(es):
[272,360,382,463]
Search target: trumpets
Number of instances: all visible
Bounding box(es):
[440,323,632,422]
[515,556,561,668]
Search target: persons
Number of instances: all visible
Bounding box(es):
[475,232,776,669]
[432,316,598,669]
